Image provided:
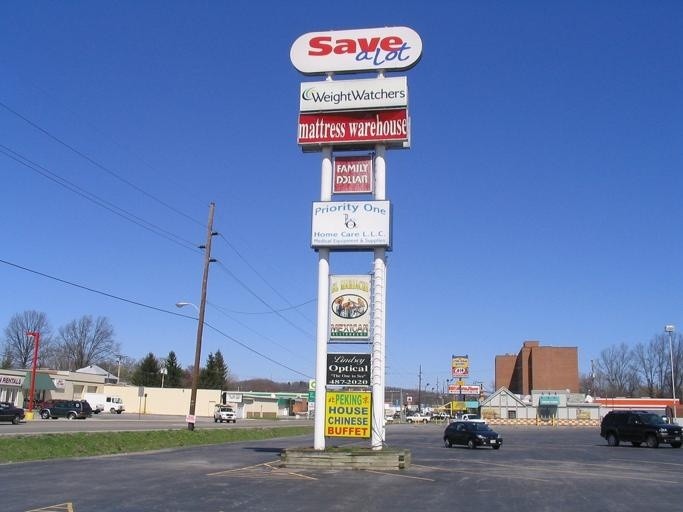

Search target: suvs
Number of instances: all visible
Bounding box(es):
[600,410,683,450]
[443,422,505,451]
[35,398,93,421]
[214,404,238,424]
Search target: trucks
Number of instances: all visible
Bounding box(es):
[83,392,126,415]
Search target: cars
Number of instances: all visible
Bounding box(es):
[384,409,489,426]
[0,402,25,426]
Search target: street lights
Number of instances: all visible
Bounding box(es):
[416,364,455,413]
[114,353,130,383]
[664,324,678,424]
[174,301,206,432]
[159,357,172,388]
[26,331,40,423]
